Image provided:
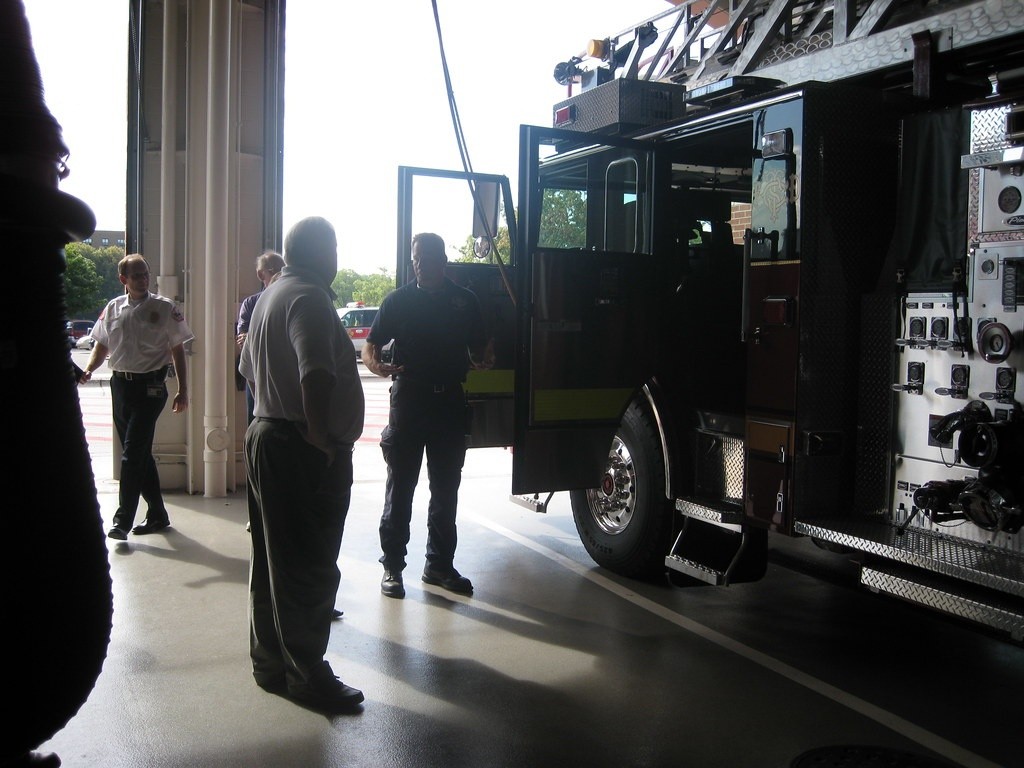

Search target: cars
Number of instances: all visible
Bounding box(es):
[336,302,395,363]
[66,320,95,344]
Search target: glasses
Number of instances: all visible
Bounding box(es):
[264,265,280,274]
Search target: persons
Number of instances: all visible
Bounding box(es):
[80,253,194,540]
[361,233,498,596]
[235,215,365,707]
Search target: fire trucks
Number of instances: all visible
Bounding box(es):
[395,1,1024,645]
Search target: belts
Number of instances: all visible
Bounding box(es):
[395,375,463,393]
[114,371,156,382]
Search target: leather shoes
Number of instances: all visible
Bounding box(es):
[132,506,170,534]
[108,522,128,540]
[254,654,365,710]
[423,561,474,591]
[332,607,343,620]
[381,568,405,599]
